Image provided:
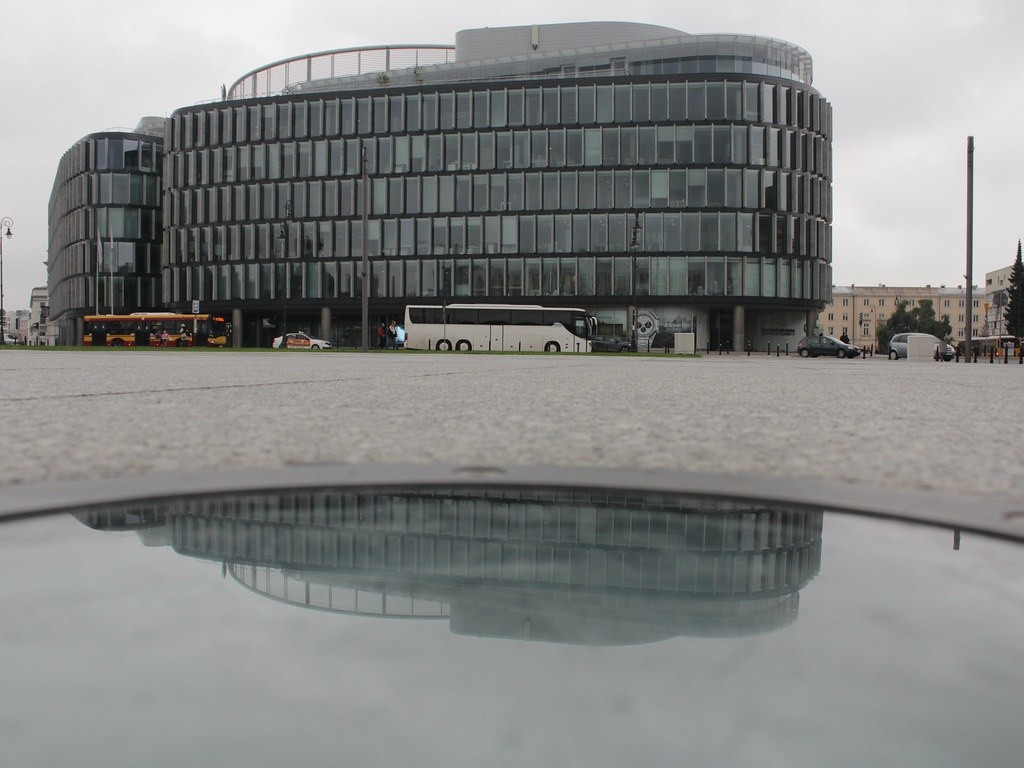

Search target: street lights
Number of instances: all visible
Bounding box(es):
[1,217,14,345]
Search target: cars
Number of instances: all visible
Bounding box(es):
[796,334,860,359]
[272,330,333,350]
[888,332,956,362]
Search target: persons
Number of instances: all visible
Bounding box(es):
[388,321,399,350]
[279,328,288,348]
[162,331,168,346]
[840,333,849,344]
[179,324,191,347]
[377,323,386,350]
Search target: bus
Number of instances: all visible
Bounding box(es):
[401,303,597,355]
[82,311,227,347]
[958,335,1021,359]
